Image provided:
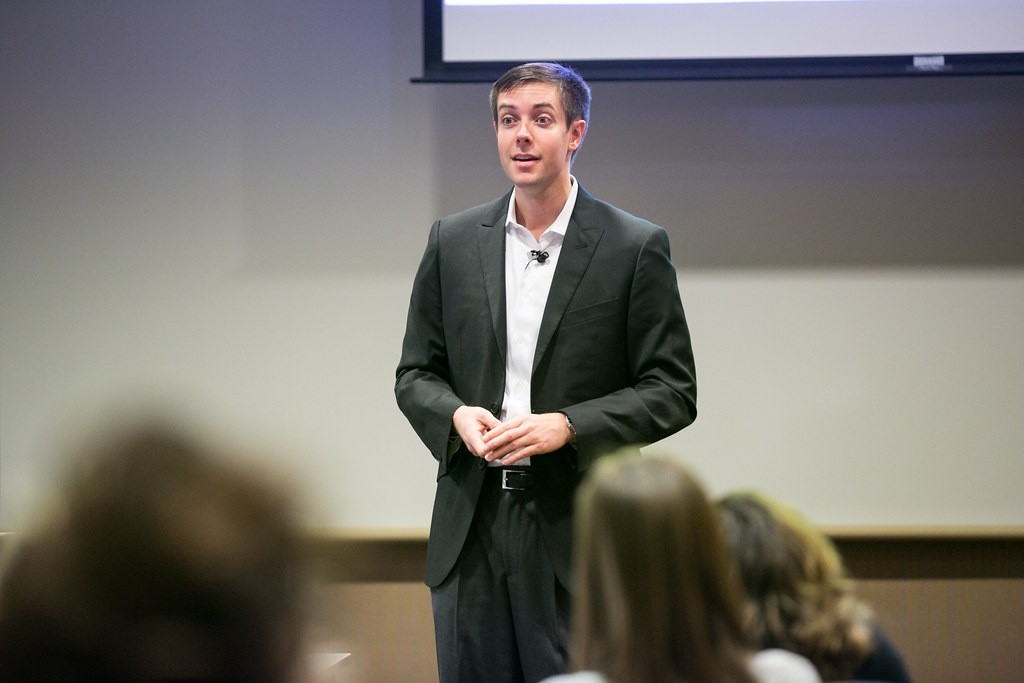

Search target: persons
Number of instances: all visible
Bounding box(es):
[393,60,699,683]
[711,487,915,682]
[1,412,318,683]
[540,443,824,683]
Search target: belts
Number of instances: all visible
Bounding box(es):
[487,467,528,493]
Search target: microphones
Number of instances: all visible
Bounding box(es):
[537,252,549,263]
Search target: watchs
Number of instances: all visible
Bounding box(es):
[566,416,577,436]
